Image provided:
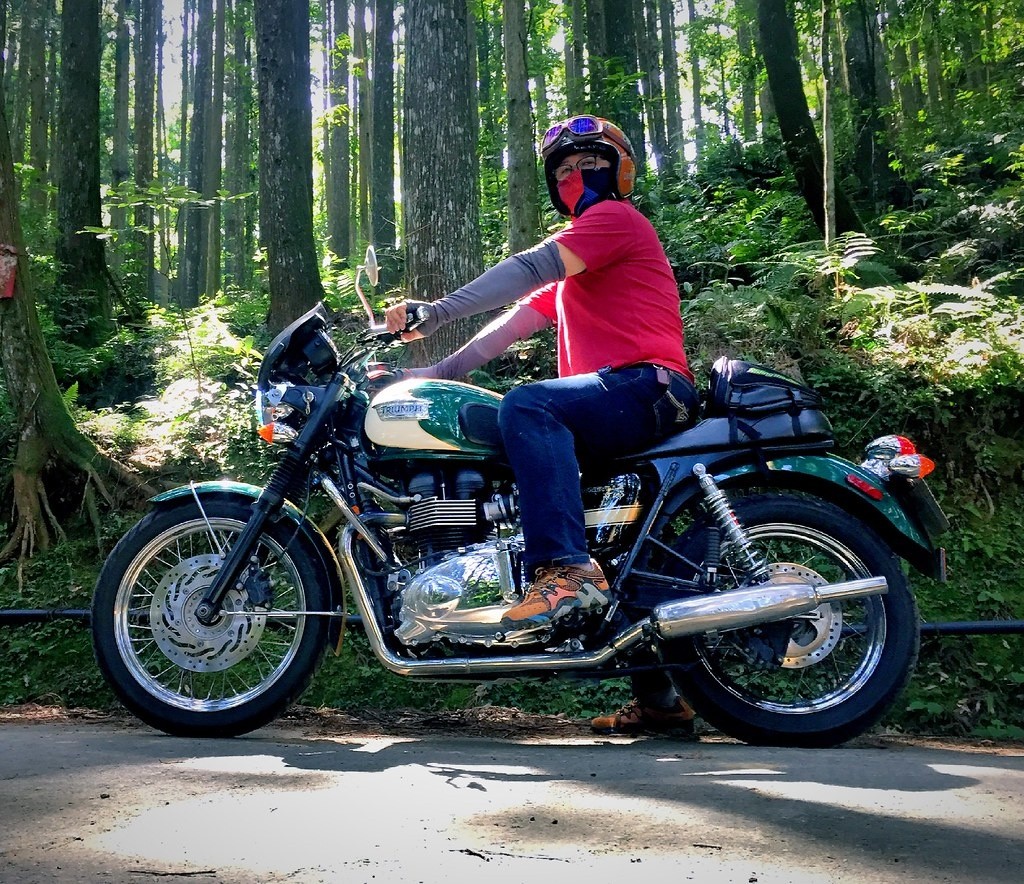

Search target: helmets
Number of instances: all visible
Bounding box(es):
[542,116,636,198]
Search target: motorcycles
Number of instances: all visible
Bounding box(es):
[89,243,953,750]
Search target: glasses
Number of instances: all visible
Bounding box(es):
[541,115,603,158]
[551,154,599,181]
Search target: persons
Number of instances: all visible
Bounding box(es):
[386,114,697,631]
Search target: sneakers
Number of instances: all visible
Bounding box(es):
[501,558,610,630]
[590,697,696,736]
